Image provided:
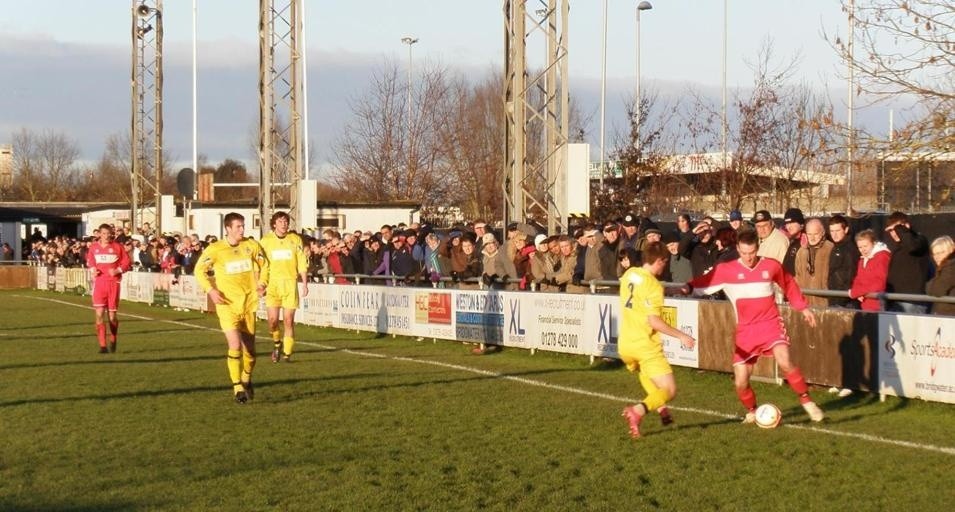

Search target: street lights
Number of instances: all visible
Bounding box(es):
[401,35,421,201]
[633,0,656,208]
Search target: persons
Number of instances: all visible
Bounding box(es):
[108,221,220,311]
[193,212,271,405]
[252,210,309,363]
[680,227,828,426]
[292,219,519,290]
[615,241,698,442]
[520,207,955,316]
[0,227,101,269]
[86,223,131,354]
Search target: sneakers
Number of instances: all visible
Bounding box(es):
[800,395,825,421]
[742,409,756,423]
[661,408,673,424]
[624,407,641,437]
[237,382,253,403]
[271,343,282,362]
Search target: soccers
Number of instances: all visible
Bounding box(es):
[755,403,783,429]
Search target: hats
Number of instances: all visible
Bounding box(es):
[622,213,636,226]
[482,233,494,246]
[730,211,741,221]
[784,209,805,224]
[644,223,661,234]
[535,234,549,248]
[751,211,770,222]
